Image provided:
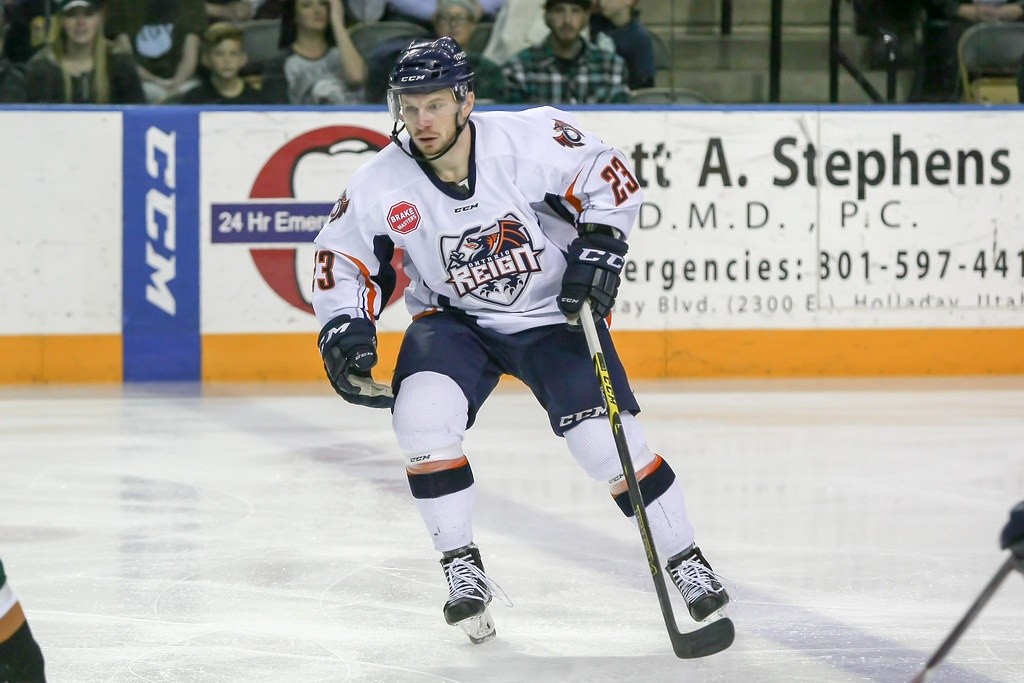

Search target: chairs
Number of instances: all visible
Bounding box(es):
[469,22,491,54]
[236,18,283,58]
[349,21,427,53]
[626,85,712,108]
[957,21,1024,103]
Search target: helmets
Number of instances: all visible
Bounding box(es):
[387,36,475,123]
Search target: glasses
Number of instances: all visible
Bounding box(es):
[438,13,473,22]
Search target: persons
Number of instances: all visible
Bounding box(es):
[0,0,657,105]
[852,0,1024,103]
[997,498,1024,588]
[311,35,730,644]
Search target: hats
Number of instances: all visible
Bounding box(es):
[545,0,591,11]
[59,0,99,12]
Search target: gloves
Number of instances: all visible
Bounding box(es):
[556,234,629,332]
[1000,502,1024,576]
[318,314,394,408]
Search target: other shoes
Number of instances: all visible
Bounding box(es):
[871,38,917,71]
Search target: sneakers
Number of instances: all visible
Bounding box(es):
[665,547,729,623]
[439,544,513,644]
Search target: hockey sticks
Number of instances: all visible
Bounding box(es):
[906,552,1018,683]
[573,302,736,661]
